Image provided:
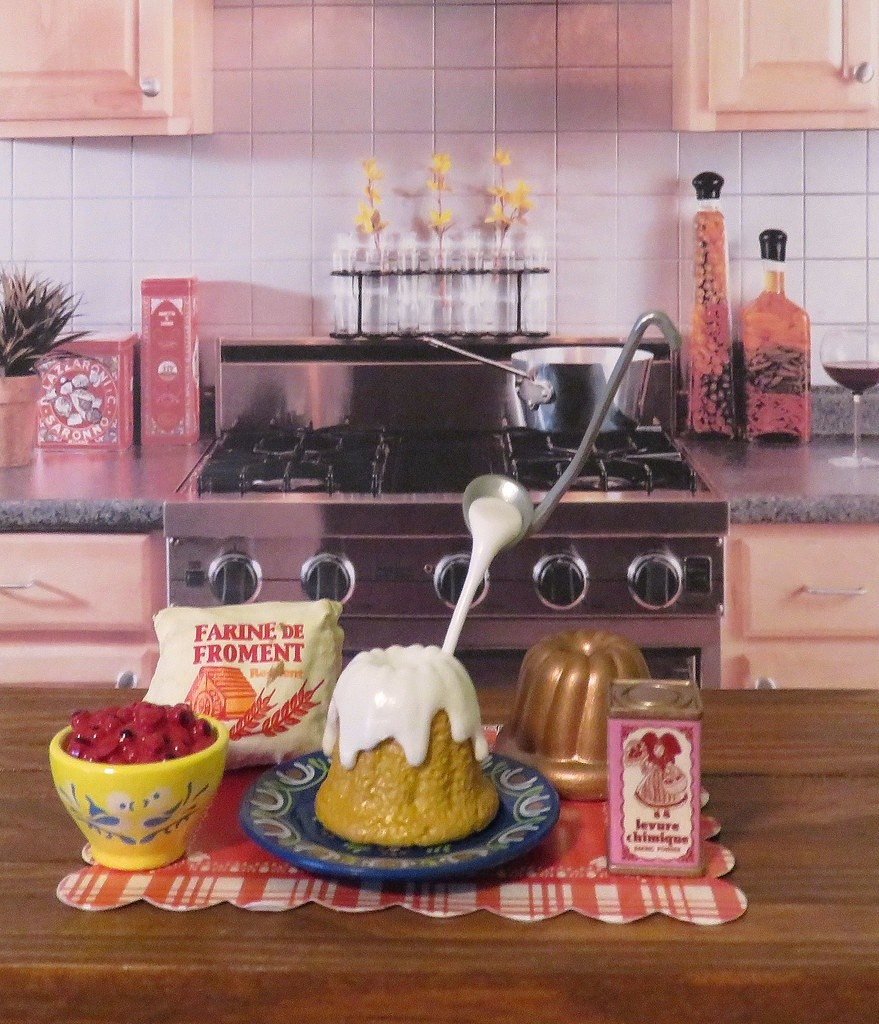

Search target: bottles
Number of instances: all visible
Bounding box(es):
[688,170,741,440]
[740,229,814,450]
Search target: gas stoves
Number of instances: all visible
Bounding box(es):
[165,414,729,620]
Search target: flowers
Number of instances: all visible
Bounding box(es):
[427,152,459,310]
[352,156,392,257]
[483,148,534,283]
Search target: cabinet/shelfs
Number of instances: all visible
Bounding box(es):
[0,531,165,689]
[673,0,879,132]
[719,521,878,690]
[1,2,214,140]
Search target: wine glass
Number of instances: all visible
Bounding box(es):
[818,325,879,472]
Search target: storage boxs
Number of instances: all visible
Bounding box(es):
[141,277,201,447]
[41,330,139,452]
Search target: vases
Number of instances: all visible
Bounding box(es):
[400,237,473,334]
[473,235,547,335]
[333,237,400,336]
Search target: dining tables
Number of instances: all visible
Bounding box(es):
[0,683,879,1024]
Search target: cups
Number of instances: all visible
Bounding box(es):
[50,711,230,877]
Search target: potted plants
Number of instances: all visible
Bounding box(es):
[0,260,97,467]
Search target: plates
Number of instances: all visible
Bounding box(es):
[237,746,564,882]
